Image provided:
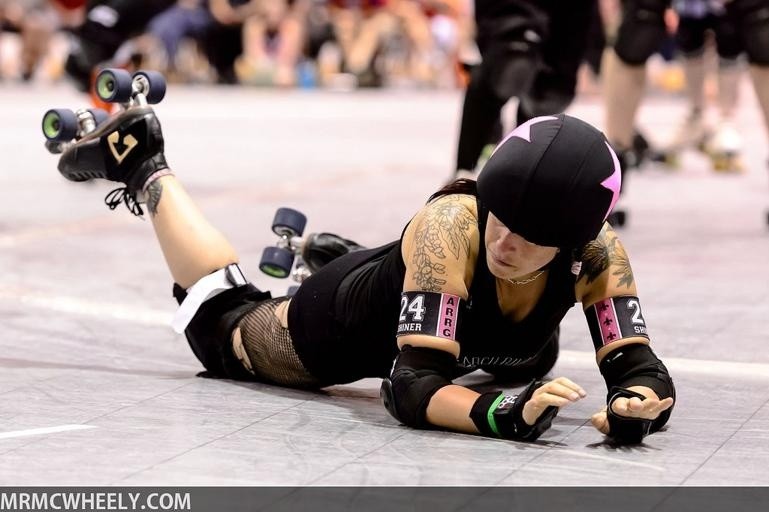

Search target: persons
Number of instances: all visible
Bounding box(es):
[455,0,602,180]
[35,65,676,448]
[705,0,768,176]
[661,0,730,155]
[1,1,768,99]
[596,0,676,175]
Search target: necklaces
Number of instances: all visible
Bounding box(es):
[505,273,544,287]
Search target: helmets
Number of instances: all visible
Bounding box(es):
[477,115,624,250]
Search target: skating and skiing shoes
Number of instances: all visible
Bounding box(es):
[258,207,367,281]
[613,130,748,174]
[42,68,169,190]
